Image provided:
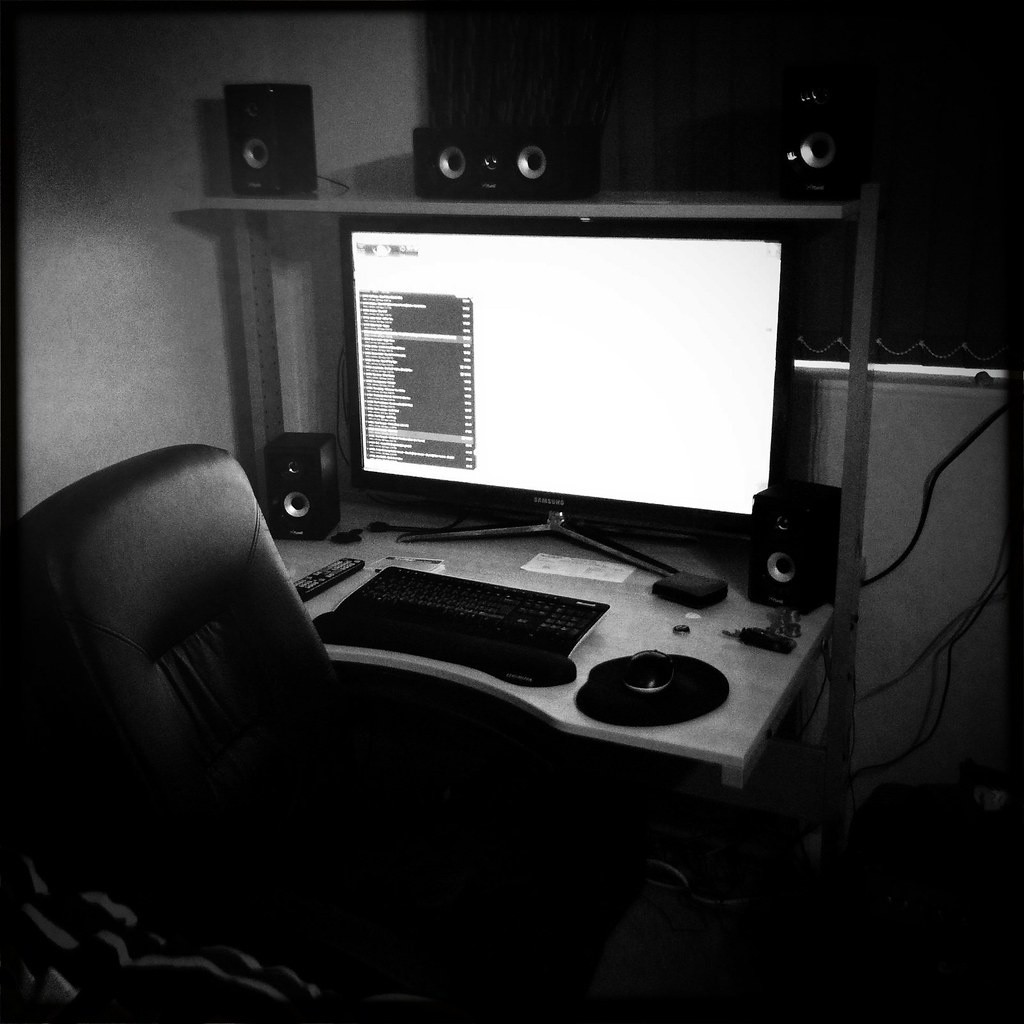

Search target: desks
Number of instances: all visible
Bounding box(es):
[157,181,888,928]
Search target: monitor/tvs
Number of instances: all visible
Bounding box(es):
[334,212,790,537]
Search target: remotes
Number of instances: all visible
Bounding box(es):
[292,558,364,602]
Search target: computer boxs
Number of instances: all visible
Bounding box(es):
[659,737,825,995]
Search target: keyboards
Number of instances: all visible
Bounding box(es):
[351,567,604,650]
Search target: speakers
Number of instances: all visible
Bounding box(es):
[748,480,842,615]
[264,432,340,540]
[412,127,600,201]
[224,84,318,195]
[778,69,860,196]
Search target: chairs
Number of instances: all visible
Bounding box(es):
[18,444,651,997]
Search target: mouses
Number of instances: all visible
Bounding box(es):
[620,649,678,696]
